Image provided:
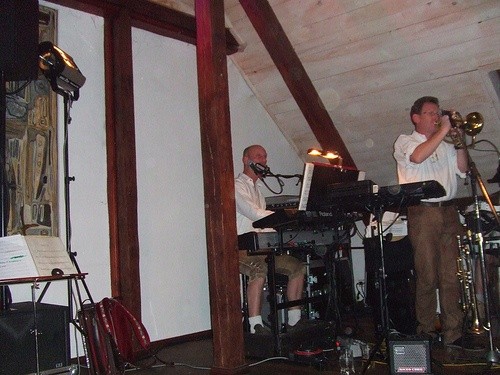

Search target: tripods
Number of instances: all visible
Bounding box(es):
[360,208,444,375]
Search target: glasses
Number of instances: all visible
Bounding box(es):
[418,110,442,117]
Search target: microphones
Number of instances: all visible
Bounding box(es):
[437,109,452,117]
[248,160,270,172]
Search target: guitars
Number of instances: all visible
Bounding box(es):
[67,251,126,375]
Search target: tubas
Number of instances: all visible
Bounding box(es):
[434,110,484,150]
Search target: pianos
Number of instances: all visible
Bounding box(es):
[237,209,364,357]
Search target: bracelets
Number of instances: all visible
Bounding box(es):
[457,147,464,149]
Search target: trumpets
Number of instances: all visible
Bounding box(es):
[455,234,486,335]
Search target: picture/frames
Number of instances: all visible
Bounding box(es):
[5,4,58,238]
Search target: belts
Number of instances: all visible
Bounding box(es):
[418,200,455,208]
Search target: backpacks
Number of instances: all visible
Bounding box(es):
[78,296,150,375]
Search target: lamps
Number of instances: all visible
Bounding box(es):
[307,148,344,172]
[36,41,94,321]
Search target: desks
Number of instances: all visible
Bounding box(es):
[0,273,88,375]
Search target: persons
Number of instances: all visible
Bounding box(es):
[393,95,486,352]
[234,145,306,334]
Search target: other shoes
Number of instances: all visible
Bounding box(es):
[446,335,484,351]
[253,324,271,335]
[432,336,444,346]
[287,319,305,329]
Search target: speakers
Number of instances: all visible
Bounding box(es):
[1,300,70,375]
[0,0,40,78]
